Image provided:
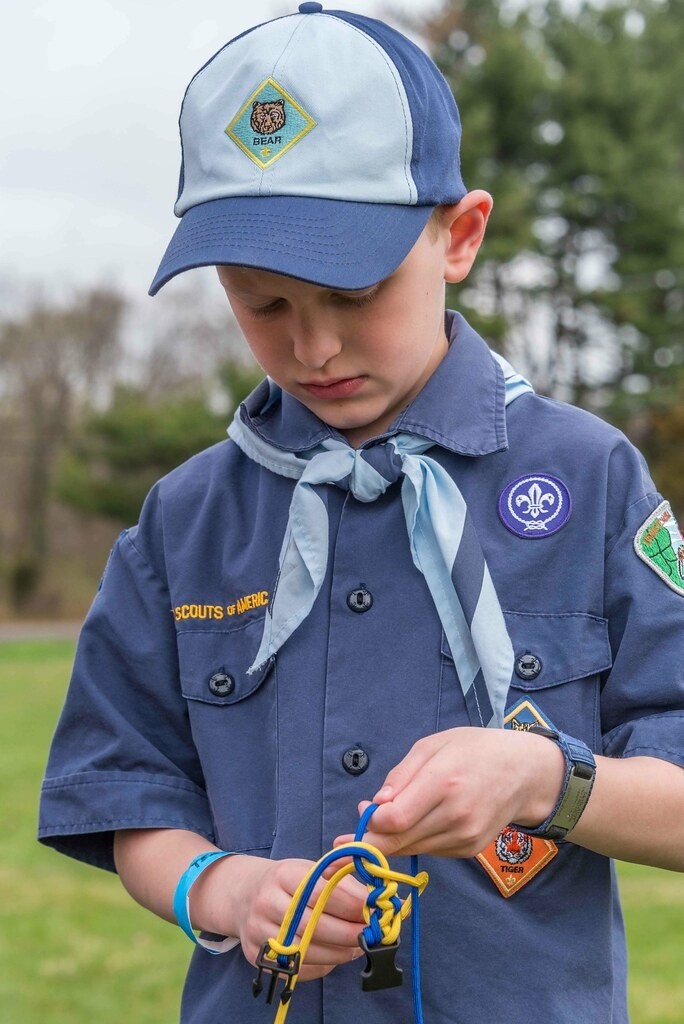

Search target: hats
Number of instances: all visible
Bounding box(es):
[149,2,469,292]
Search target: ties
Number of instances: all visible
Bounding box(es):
[228,349,536,729]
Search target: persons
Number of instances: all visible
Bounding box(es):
[36,0,684,1024]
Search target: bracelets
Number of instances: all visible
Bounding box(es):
[510,724,596,842]
[173,850,242,955]
